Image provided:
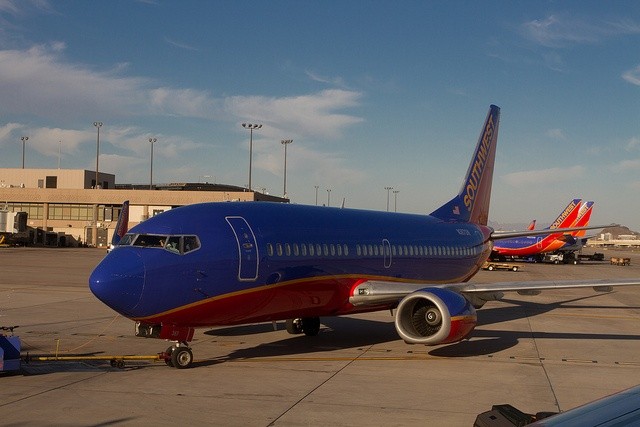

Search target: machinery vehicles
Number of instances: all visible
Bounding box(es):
[481,259,525,271]
[577,252,604,261]
[543,251,580,264]
[609,257,631,266]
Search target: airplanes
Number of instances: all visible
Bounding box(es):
[492,198,599,261]
[88,103,640,369]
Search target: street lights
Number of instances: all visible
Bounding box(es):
[314,185,319,205]
[327,189,332,206]
[147,137,157,189]
[241,123,263,191]
[280,138,293,198]
[392,190,400,212]
[21,136,29,168]
[93,121,103,188]
[384,186,393,212]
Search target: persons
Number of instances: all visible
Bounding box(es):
[159,238,170,247]
